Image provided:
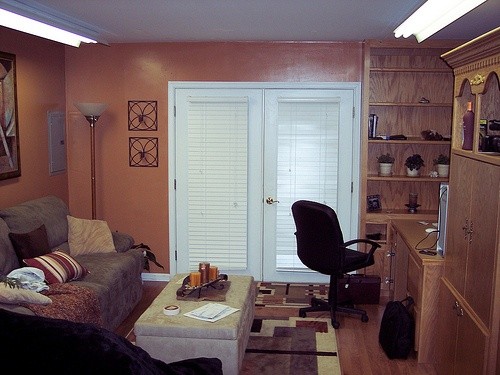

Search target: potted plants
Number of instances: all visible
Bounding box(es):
[404,154,426,177]
[434,154,450,178]
[375,152,395,176]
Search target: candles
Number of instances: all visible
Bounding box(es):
[409,192,418,205]
[189,263,218,287]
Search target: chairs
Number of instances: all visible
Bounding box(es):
[291,200,382,328]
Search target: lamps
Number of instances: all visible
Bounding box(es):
[393,0,487,45]
[0,0,112,48]
[74,102,110,219]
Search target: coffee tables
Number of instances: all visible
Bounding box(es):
[135,273,257,375]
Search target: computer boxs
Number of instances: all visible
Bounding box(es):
[435,183,449,257]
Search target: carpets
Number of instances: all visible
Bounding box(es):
[125,280,343,375]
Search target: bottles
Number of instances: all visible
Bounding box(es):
[461,101,475,150]
[369,108,378,137]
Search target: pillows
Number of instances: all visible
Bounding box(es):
[0,215,116,306]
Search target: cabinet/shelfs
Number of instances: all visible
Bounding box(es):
[357,27,500,375]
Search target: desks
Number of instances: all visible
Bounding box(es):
[385,219,443,364]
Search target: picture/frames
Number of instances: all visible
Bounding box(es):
[367,193,382,211]
[0,49,22,180]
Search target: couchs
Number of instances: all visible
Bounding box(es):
[0,307,225,375]
[0,194,145,332]
